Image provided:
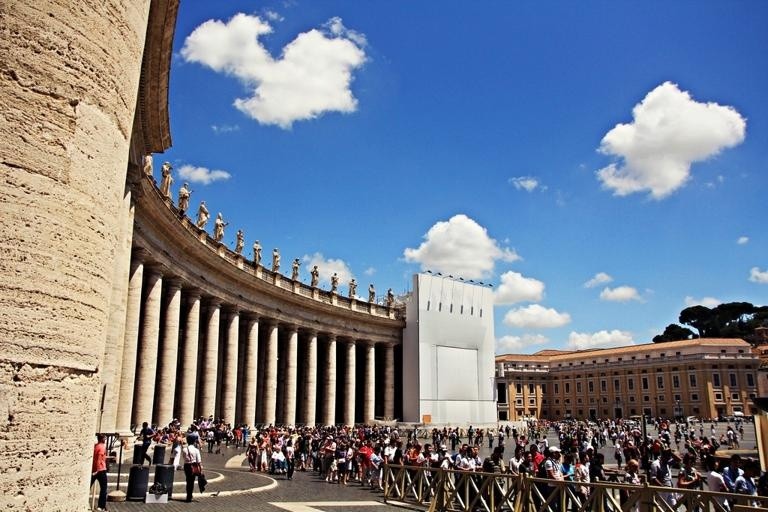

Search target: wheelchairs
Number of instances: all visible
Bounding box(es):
[267,459,287,475]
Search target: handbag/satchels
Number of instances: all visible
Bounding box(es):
[149,482,167,494]
[191,465,201,476]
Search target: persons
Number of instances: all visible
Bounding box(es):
[91,434,110,512]
[141,154,394,307]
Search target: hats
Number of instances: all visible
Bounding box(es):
[586,445,594,450]
[548,445,562,453]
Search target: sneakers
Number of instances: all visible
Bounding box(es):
[248,466,372,486]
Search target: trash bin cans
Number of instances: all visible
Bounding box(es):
[126,443,174,502]
